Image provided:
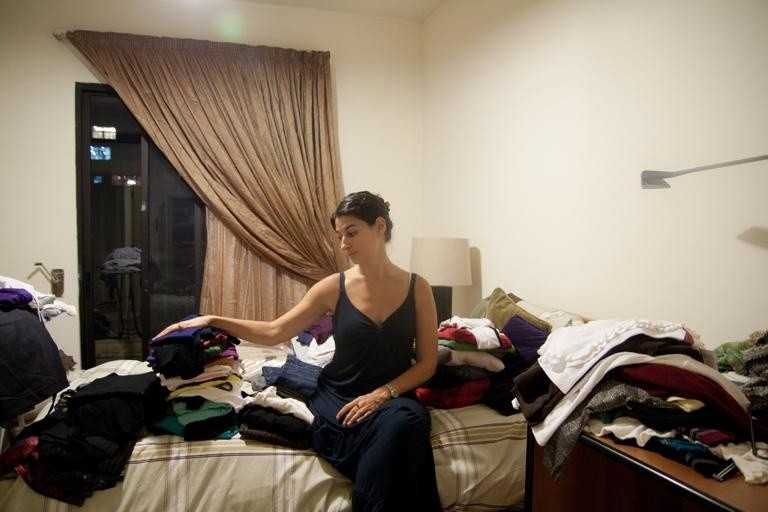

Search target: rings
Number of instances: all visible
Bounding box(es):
[357,405,360,408]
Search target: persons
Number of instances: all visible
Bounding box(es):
[152,189,442,512]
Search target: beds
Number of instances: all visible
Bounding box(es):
[0,339,528,512]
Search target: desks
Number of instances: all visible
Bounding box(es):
[524,421,767,511]
[101,268,143,339]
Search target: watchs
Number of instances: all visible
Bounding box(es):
[386,383,400,398]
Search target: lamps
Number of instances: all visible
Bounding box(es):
[409,237,473,328]
[641,155,768,190]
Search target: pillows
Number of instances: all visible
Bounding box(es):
[468,287,584,366]
